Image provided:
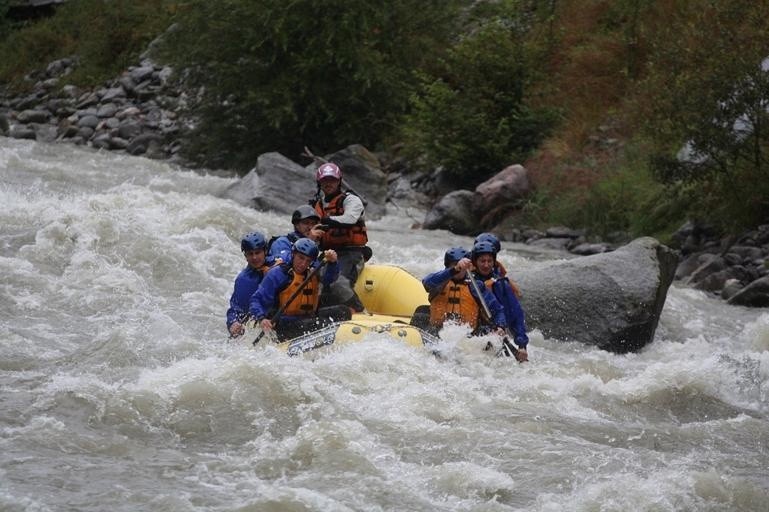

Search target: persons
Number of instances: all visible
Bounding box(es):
[395,232,529,363]
[227,163,372,344]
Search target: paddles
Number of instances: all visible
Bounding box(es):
[465,268,529,363]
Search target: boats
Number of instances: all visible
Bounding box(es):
[277,265,434,358]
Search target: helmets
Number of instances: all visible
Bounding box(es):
[444,247,470,265]
[314,163,343,182]
[291,238,319,257]
[241,232,267,251]
[474,232,501,252]
[471,241,497,258]
[292,205,321,223]
